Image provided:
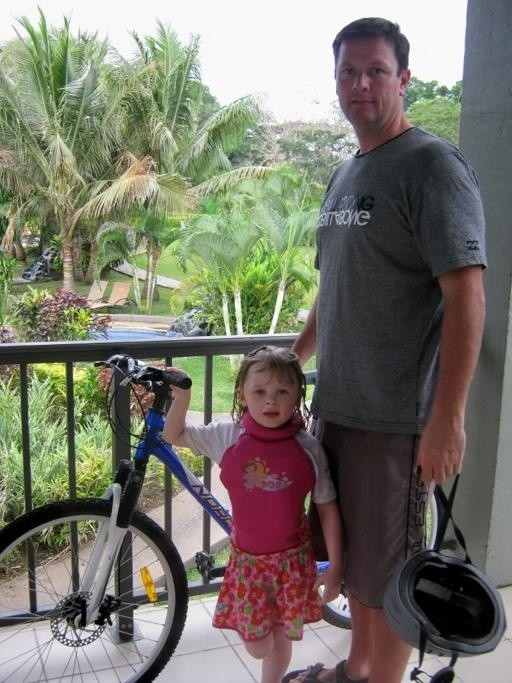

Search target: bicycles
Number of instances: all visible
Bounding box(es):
[0,348,456,682]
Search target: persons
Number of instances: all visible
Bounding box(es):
[162,343,345,683]
[280,16,489,682]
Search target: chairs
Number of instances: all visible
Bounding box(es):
[78,280,137,312]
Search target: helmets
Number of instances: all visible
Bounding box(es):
[383,549,506,658]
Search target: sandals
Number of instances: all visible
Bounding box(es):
[282,661,368,683]
